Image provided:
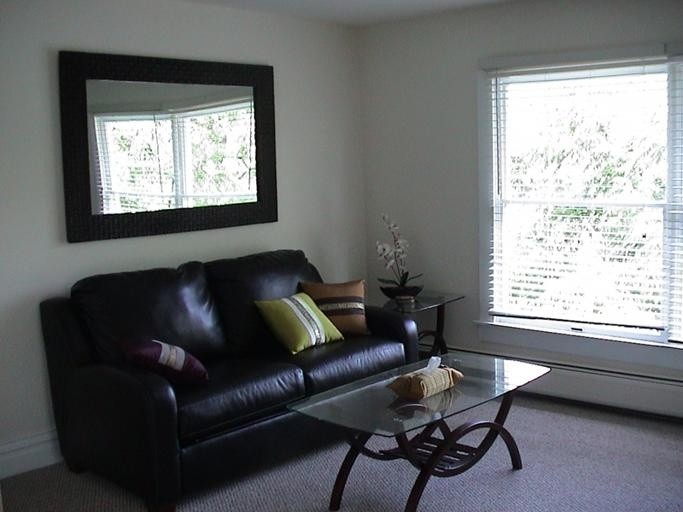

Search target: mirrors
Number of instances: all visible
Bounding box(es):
[57,48,279,243]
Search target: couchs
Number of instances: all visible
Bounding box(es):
[38,248,421,511]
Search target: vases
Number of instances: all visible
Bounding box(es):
[379,284,420,300]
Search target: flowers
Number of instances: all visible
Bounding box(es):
[368,208,425,288]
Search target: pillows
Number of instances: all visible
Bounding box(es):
[113,326,208,389]
[296,276,370,338]
[251,288,344,356]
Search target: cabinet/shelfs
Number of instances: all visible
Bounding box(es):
[363,280,465,354]
